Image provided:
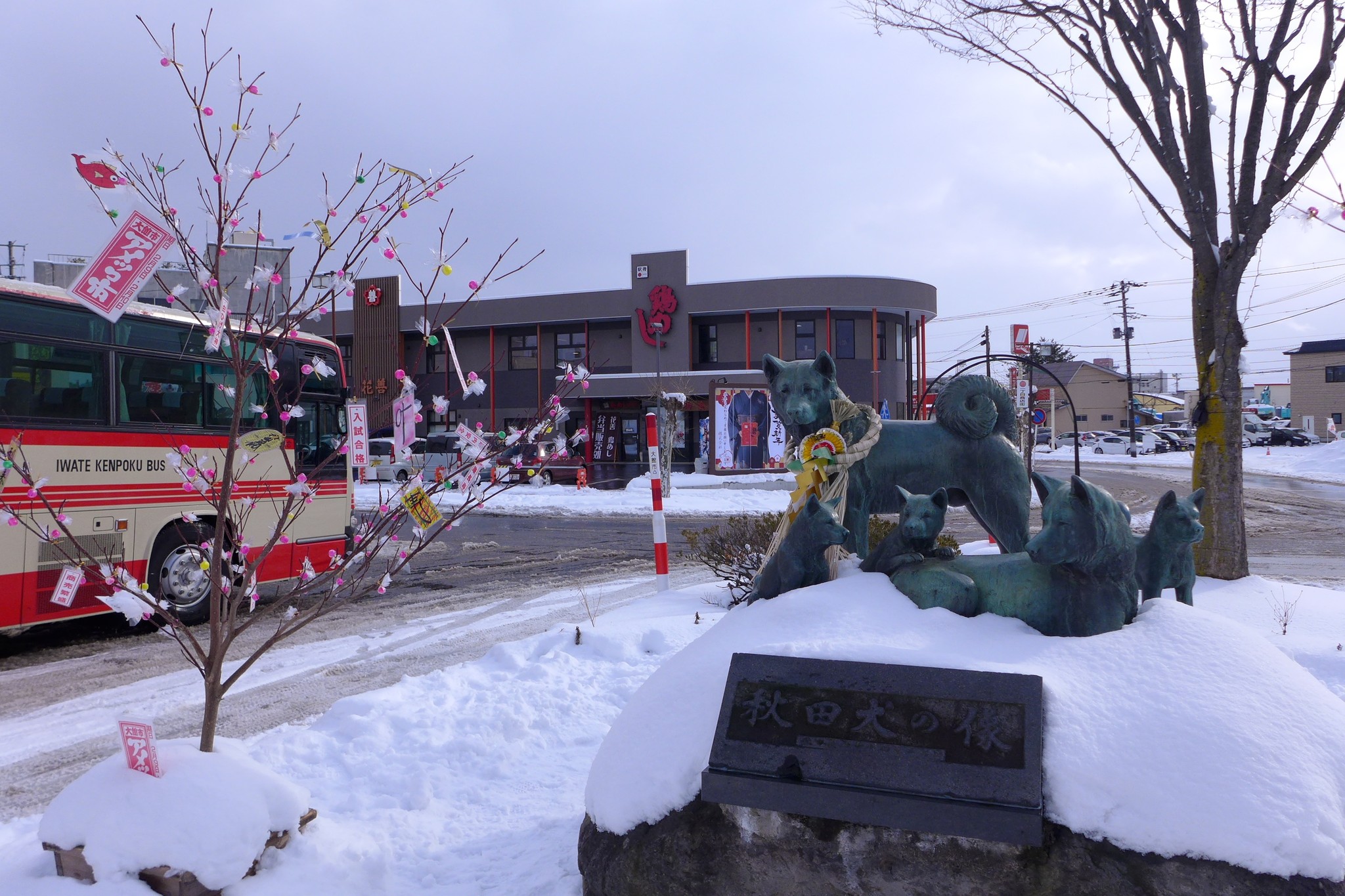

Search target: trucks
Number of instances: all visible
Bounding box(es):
[1240,412,1272,446]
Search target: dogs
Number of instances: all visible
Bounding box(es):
[747,350,1207,637]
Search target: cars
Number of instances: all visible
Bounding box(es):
[495,440,587,486]
[1087,419,1198,455]
[1015,427,1052,447]
[1048,431,1096,451]
[1241,434,1251,448]
[1334,431,1345,442]
[297,432,339,465]
[1263,419,1321,447]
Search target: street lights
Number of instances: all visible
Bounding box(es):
[649,322,663,470]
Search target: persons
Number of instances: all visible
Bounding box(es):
[0,339,211,427]
[728,390,771,469]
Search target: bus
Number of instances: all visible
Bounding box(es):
[0,274,360,642]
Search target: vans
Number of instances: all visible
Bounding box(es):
[356,437,430,483]
[420,431,508,486]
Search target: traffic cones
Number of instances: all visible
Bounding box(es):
[1266,447,1270,455]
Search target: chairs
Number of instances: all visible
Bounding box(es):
[0,382,215,428]
